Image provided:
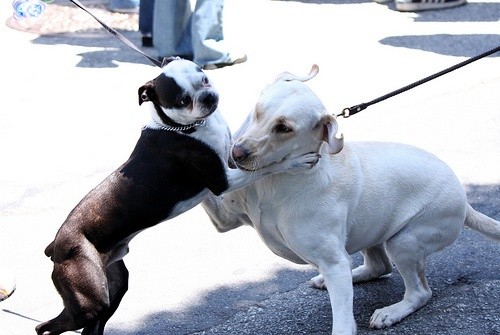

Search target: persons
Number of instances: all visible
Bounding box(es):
[376,0,467,13]
[137,1,154,47]
[151,0,247,70]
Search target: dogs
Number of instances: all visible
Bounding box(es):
[34,55,322,335]
[202,65,500,335]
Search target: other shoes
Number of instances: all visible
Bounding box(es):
[160,54,191,63]
[203,50,248,70]
[139,34,154,46]
[396,0,466,12]
